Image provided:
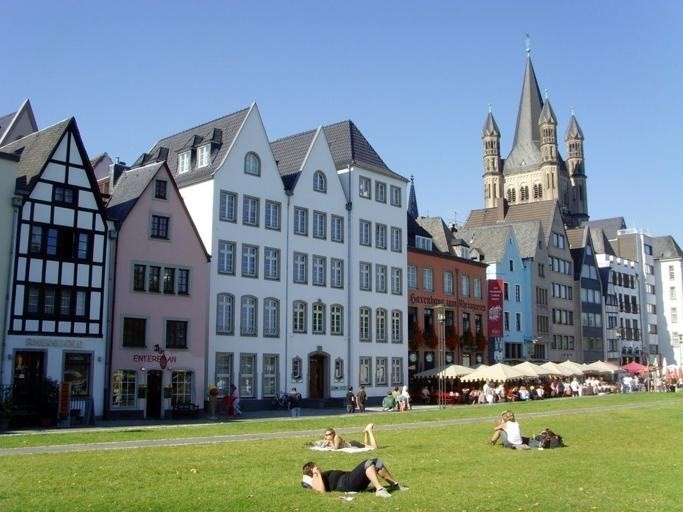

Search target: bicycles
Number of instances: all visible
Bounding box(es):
[271,391,290,410]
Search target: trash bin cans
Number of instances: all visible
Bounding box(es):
[670,386,675,392]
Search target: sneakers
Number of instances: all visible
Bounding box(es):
[364,424,373,432]
[391,483,409,490]
[375,487,392,497]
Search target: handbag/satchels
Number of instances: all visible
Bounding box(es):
[536,428,563,448]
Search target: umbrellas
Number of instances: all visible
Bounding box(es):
[413,360,654,391]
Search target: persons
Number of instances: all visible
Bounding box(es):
[300,457,410,499]
[287,387,304,417]
[320,423,378,451]
[229,383,243,412]
[112,384,122,404]
[484,408,524,449]
[230,386,242,417]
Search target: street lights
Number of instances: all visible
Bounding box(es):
[429,303,449,411]
